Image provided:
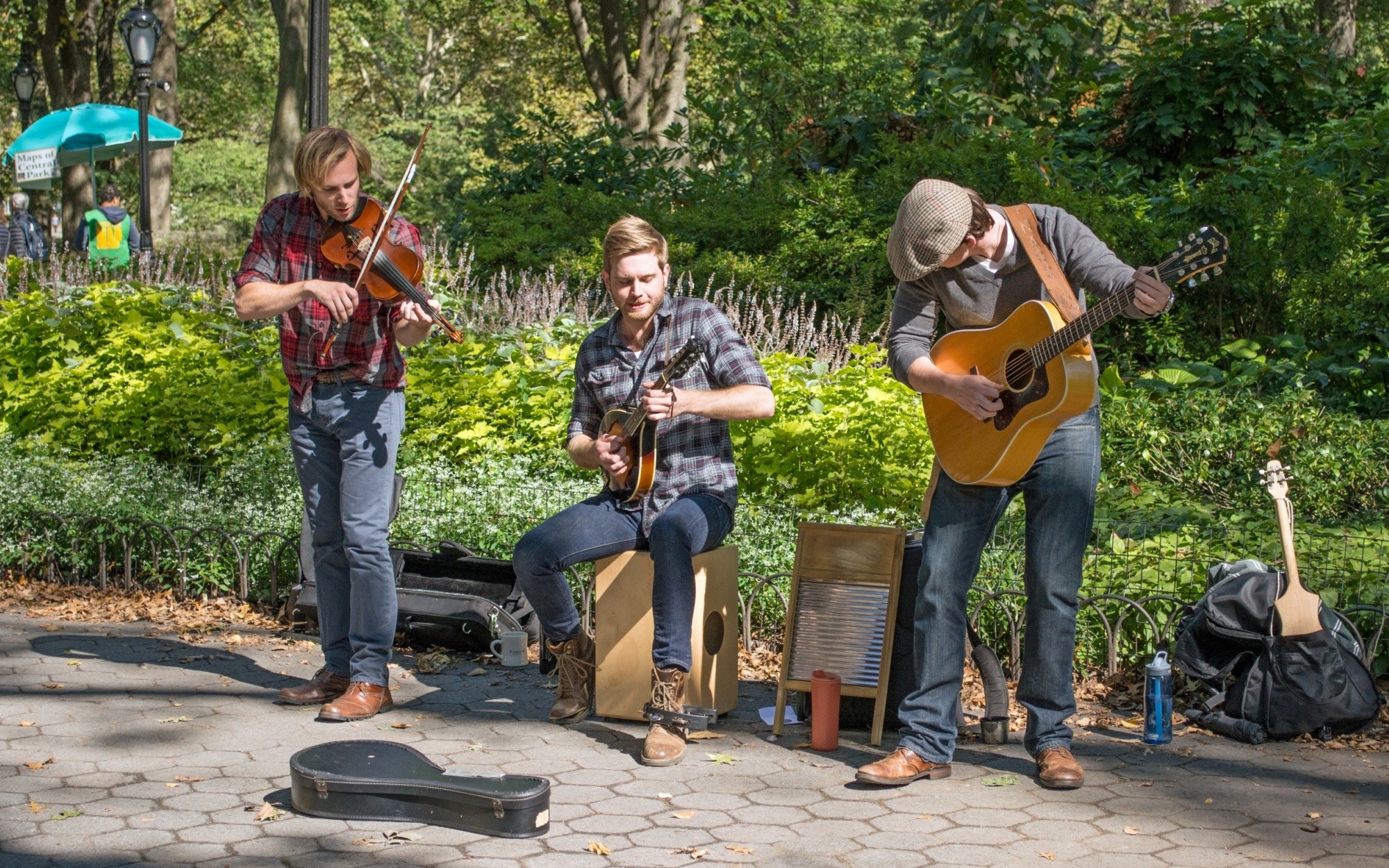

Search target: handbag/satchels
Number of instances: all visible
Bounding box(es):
[1170,558,1382,739]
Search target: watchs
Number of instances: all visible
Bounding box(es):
[1157,284,1174,317]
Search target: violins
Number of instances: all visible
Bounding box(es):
[320,198,466,346]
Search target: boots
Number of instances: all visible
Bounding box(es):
[640,667,687,766]
[545,627,596,723]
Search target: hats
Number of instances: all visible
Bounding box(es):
[887,179,972,282]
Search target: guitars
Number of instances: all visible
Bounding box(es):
[596,334,707,505]
[1258,460,1324,636]
[922,223,1230,489]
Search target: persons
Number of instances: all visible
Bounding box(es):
[856,180,1174,790]
[511,216,774,768]
[234,127,439,719]
[0,184,141,275]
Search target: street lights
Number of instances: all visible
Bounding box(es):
[117,0,167,252]
[9,44,41,215]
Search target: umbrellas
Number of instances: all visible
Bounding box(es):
[3,102,183,213]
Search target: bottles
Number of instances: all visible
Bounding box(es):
[539,621,559,675]
[1144,651,1173,744]
[811,669,841,751]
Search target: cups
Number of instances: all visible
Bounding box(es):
[490,631,529,666]
[979,716,1010,745]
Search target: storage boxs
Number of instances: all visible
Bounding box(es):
[593,544,749,726]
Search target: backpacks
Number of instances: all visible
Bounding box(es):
[18,216,48,263]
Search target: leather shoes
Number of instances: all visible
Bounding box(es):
[855,748,952,786]
[320,682,394,722]
[1036,747,1083,788]
[278,669,350,706]
